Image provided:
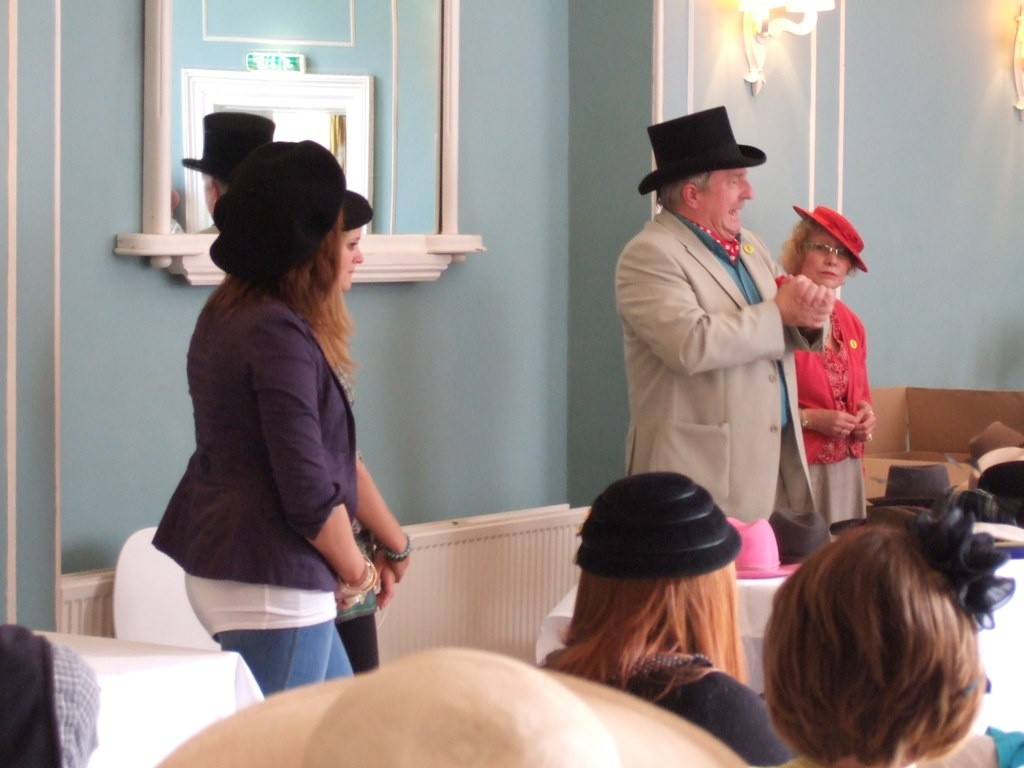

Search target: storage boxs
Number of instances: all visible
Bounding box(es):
[865,384,1024,502]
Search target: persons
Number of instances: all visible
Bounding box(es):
[152,142,379,694]
[773,205,875,541]
[535,471,793,768]
[182,111,277,234]
[763,494,1024,768]
[616,104,837,521]
[334,191,412,674]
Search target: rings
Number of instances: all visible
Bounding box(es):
[842,429,846,436]
[866,433,873,440]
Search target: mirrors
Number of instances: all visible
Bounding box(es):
[116,0,486,286]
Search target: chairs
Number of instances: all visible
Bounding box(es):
[112,528,222,651]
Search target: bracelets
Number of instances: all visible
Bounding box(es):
[338,556,378,592]
[385,534,411,562]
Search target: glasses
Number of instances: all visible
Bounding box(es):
[799,238,851,261]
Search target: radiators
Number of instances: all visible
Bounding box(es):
[61,501,593,668]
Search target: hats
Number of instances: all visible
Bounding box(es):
[793,200,869,273]
[577,471,740,577]
[637,106,767,195]
[726,509,867,579]
[209,141,347,280]
[182,113,275,179]
[869,464,955,503]
[342,190,374,231]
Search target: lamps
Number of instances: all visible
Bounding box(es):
[742,0,836,98]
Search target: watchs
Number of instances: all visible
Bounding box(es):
[801,409,809,429]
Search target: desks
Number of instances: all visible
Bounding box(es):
[35,630,266,768]
[537,577,790,696]
